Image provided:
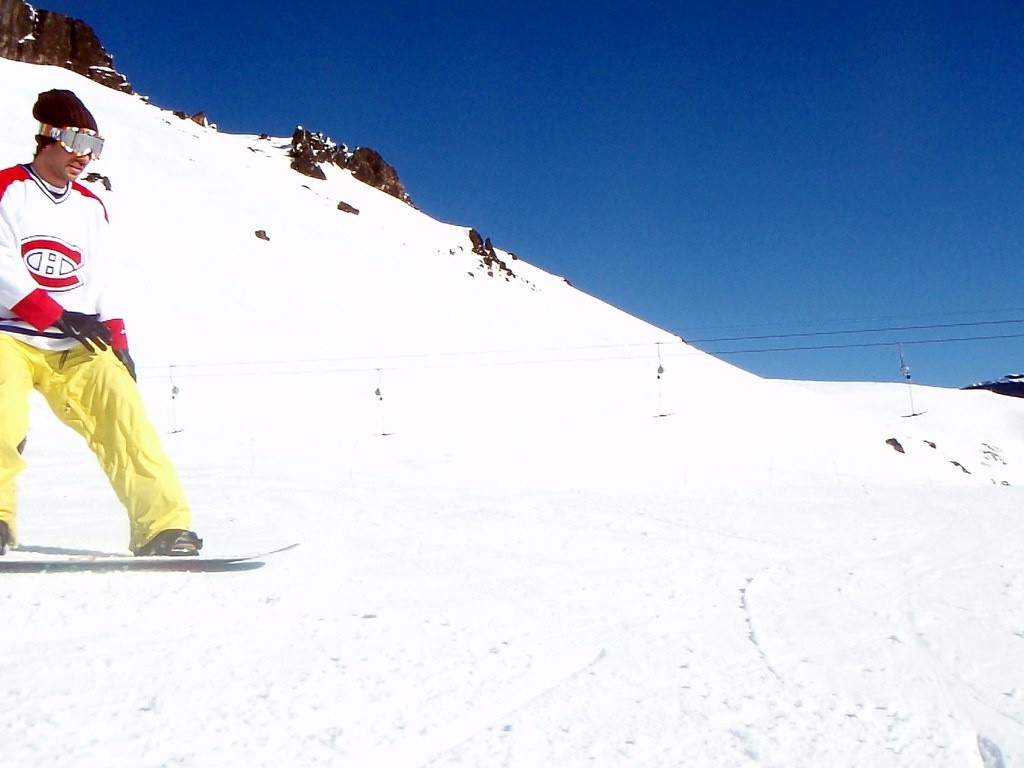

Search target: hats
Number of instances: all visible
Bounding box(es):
[33,89,99,147]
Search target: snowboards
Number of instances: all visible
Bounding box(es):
[1,542,301,566]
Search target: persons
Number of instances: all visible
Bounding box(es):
[0,89,204,557]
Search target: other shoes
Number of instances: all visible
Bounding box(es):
[131,530,203,556]
[0,517,13,554]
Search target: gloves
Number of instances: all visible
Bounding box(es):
[53,309,114,354]
[116,349,136,383]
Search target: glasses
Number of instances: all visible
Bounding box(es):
[36,121,105,161]
